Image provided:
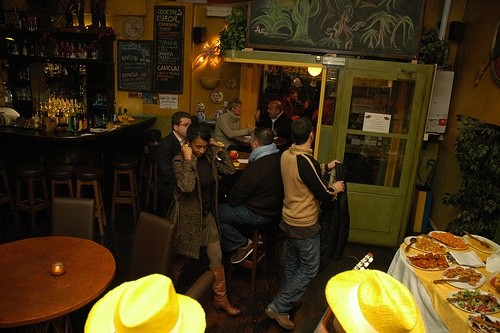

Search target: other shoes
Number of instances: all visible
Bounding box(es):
[265,306,295,330]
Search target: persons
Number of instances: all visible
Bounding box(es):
[152,76,336,316]
[263,116,345,330]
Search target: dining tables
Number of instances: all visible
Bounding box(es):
[386,231,500,333]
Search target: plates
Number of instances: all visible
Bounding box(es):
[407,253,452,270]
[463,234,500,254]
[404,235,448,255]
[442,266,486,289]
[467,313,500,333]
[448,290,500,315]
[428,230,468,249]
[488,272,500,289]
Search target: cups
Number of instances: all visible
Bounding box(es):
[49,261,65,275]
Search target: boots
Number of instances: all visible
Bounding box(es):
[210,264,241,316]
[170,269,182,286]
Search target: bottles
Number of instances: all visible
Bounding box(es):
[5,16,107,131]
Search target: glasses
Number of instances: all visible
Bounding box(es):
[176,124,188,127]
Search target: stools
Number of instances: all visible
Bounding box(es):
[49,166,74,203]
[0,167,14,212]
[111,160,138,225]
[139,152,159,209]
[14,164,50,235]
[227,222,279,290]
[74,166,107,237]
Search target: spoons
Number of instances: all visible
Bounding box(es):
[405,238,417,251]
[464,230,490,249]
[433,277,470,284]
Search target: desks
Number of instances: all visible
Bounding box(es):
[226,150,250,171]
[236,133,288,149]
[0,237,116,333]
[0,117,155,208]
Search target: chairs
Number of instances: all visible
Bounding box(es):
[51,195,96,241]
[124,212,176,276]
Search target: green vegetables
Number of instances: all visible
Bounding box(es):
[452,290,500,312]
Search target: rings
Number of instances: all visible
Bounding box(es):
[185,143,189,147]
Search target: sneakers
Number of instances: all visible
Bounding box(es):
[258,234,262,245]
[230,239,253,263]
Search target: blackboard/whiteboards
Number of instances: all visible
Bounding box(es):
[117,39,153,93]
[154,4,184,94]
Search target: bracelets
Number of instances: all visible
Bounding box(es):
[325,162,332,174]
[217,141,224,149]
[331,187,338,201]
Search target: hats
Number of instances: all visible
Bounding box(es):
[325,269,427,333]
[85,274,206,333]
